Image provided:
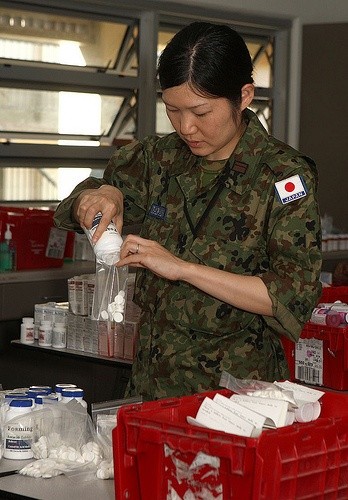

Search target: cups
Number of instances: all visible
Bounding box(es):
[249,385,321,423]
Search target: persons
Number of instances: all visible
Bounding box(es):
[51,21,324,399]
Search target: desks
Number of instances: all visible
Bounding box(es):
[0,458,115,500]
[322,250,348,282]
[11,340,133,367]
[0,257,95,321]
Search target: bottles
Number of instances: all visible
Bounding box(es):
[38,321,52,347]
[82,211,124,266]
[309,308,340,327]
[20,317,35,344]
[0,223,16,273]
[0,383,87,459]
[317,312,348,324]
[51,322,67,349]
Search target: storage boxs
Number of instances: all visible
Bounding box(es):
[0,205,74,271]
[111,379,348,500]
[34,273,142,360]
[280,286,348,390]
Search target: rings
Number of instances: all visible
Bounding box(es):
[136,244,139,253]
